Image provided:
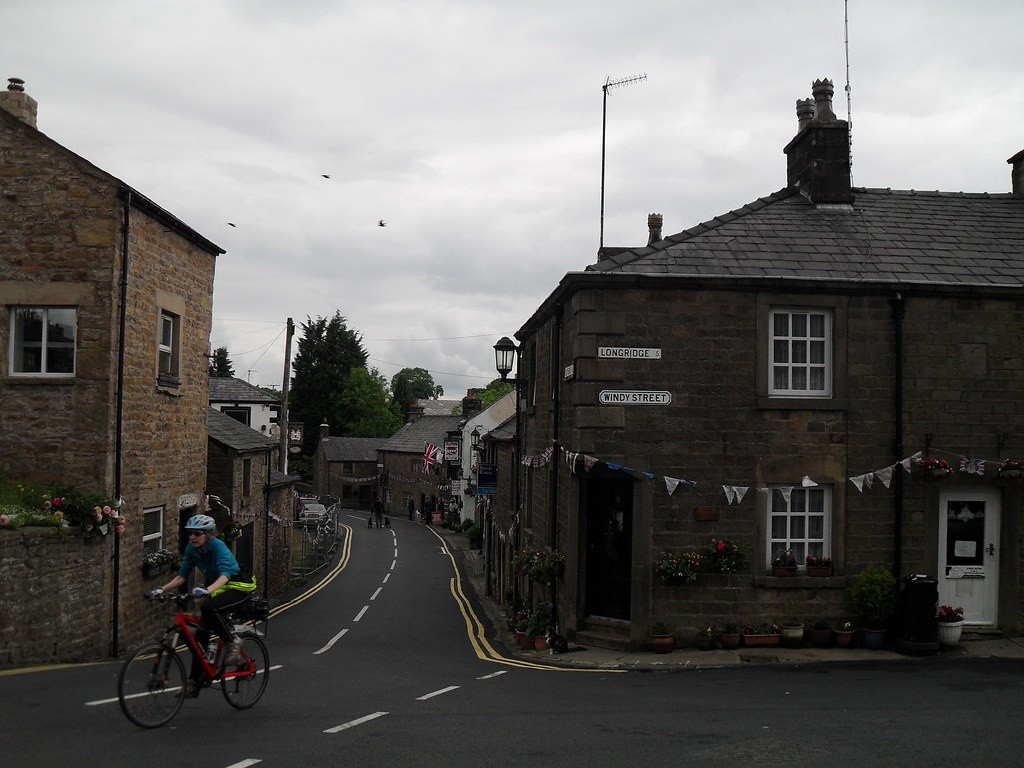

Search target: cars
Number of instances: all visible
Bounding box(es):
[298,496,320,515]
[298,504,329,528]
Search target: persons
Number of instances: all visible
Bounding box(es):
[449,499,458,531]
[408,495,415,522]
[438,499,445,523]
[423,497,434,525]
[149,515,258,698]
[370,498,387,529]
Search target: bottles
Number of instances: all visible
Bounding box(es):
[207,639,218,664]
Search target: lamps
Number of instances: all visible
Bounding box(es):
[469,427,486,452]
[493,337,530,388]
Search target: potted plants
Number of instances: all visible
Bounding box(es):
[850,564,897,650]
[719,621,741,650]
[533,601,553,652]
[779,589,857,647]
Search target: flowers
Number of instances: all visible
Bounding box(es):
[936,604,965,622]
[697,622,716,640]
[505,546,565,631]
[142,548,174,566]
[805,547,833,568]
[648,608,674,635]
[769,547,797,566]
[743,622,780,635]
[655,532,752,587]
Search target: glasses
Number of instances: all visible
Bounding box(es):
[187,531,207,537]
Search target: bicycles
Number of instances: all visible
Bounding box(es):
[116,589,274,729]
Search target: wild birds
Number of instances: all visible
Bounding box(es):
[228,223,236,227]
[378,220,386,227]
[322,175,329,178]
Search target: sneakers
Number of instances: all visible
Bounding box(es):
[175,679,201,699]
[224,634,243,666]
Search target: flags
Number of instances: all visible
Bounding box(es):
[337,474,381,482]
[565,450,598,474]
[389,474,452,490]
[268,510,294,527]
[520,446,554,468]
[421,442,439,475]
[960,457,985,476]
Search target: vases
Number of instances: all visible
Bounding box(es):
[807,566,830,576]
[141,561,173,576]
[771,565,796,576]
[649,632,674,654]
[936,620,963,646]
[743,634,781,647]
[695,637,715,650]
[506,619,533,650]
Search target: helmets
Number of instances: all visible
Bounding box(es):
[184,514,216,530]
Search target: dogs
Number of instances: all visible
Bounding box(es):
[368,517,372,528]
[384,517,391,528]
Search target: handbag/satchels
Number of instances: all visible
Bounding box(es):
[234,598,268,622]
